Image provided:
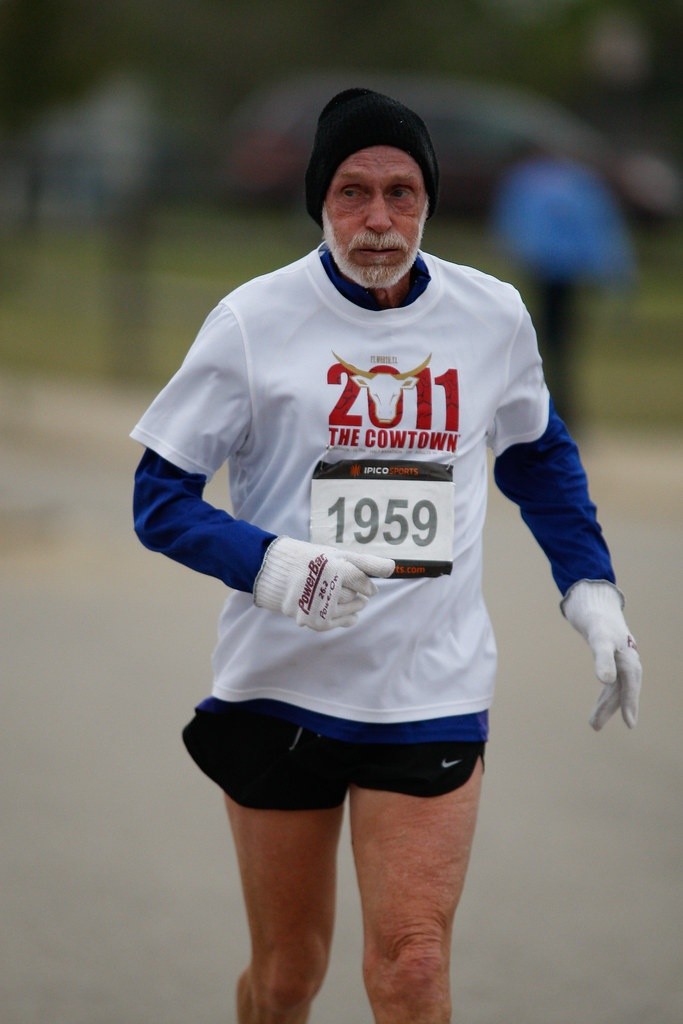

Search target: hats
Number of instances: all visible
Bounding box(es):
[306,88,438,230]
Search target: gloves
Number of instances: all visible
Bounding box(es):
[560,579,642,731]
[250,534,395,631]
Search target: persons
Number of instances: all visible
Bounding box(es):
[490,137,629,428]
[124,89,645,1023]
